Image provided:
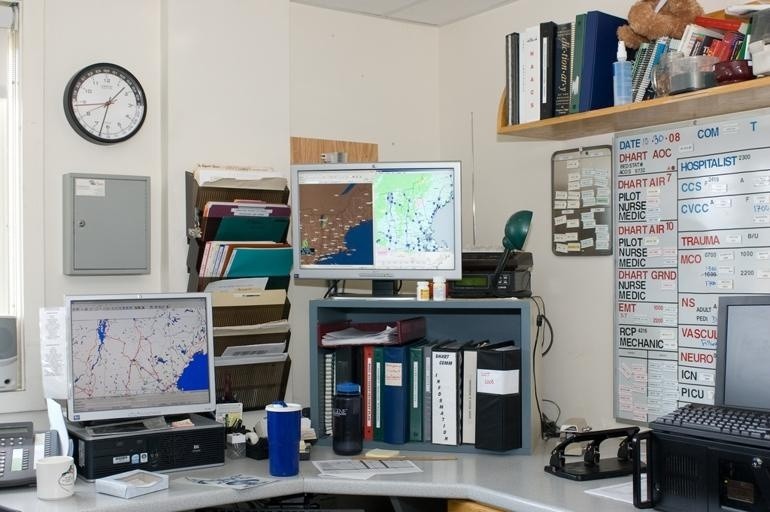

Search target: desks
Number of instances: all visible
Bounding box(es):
[1,428,660,512]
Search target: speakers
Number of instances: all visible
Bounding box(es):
[0,315,18,392]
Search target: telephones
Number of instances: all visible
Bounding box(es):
[0,422,59,488]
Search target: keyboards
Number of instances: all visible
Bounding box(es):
[648,403,770,448]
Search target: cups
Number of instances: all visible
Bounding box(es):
[650,51,681,97]
[35,455,77,500]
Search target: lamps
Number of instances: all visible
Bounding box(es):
[486,209,533,298]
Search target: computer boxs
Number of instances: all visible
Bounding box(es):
[65,413,225,483]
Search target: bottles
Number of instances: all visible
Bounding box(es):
[226,432,246,459]
[415,280,429,300]
[333,382,362,455]
[432,277,447,301]
[264,402,303,476]
[611,38,634,107]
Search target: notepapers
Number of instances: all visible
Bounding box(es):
[365,448,399,459]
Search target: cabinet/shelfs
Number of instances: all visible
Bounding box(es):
[62,172,152,276]
[308,295,541,456]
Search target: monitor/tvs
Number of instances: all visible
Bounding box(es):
[714,296,770,412]
[65,292,217,422]
[291,160,462,297]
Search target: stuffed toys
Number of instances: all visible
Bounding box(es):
[616,0,705,50]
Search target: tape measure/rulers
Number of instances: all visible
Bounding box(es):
[224,372,232,403]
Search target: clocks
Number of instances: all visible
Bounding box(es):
[62,62,149,147]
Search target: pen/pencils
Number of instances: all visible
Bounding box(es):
[225,414,245,433]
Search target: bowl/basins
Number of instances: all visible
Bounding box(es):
[714,60,756,86]
